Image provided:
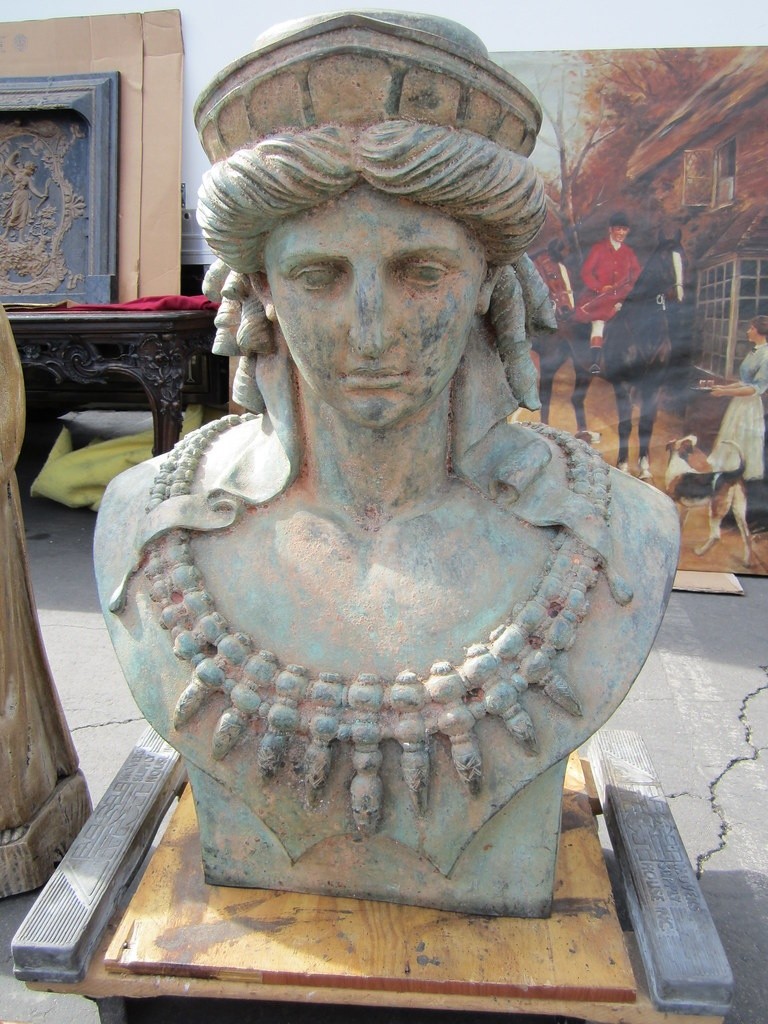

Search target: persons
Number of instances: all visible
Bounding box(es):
[0,300,79,831]
[93,13,680,919]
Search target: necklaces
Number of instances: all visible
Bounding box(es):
[142,414,610,835]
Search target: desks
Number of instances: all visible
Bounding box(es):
[8,309,230,457]
